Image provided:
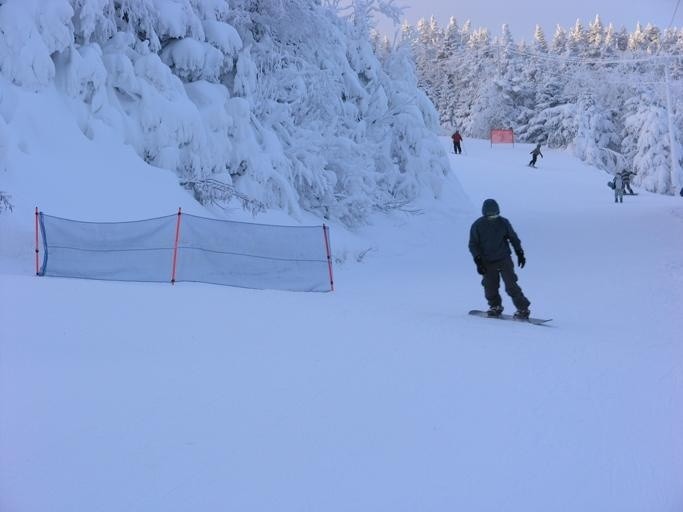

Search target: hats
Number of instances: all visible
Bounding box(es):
[482,199,500,216]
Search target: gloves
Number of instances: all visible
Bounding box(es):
[516,250,526,268]
[475,256,488,274]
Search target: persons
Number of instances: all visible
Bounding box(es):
[613,173,624,202]
[529,144,543,166]
[452,130,462,154]
[621,169,633,193]
[469,199,530,318]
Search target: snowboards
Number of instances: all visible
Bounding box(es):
[469,310,553,325]
[608,182,615,189]
[618,194,638,195]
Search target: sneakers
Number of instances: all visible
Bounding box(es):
[514,307,530,318]
[487,304,504,316]
